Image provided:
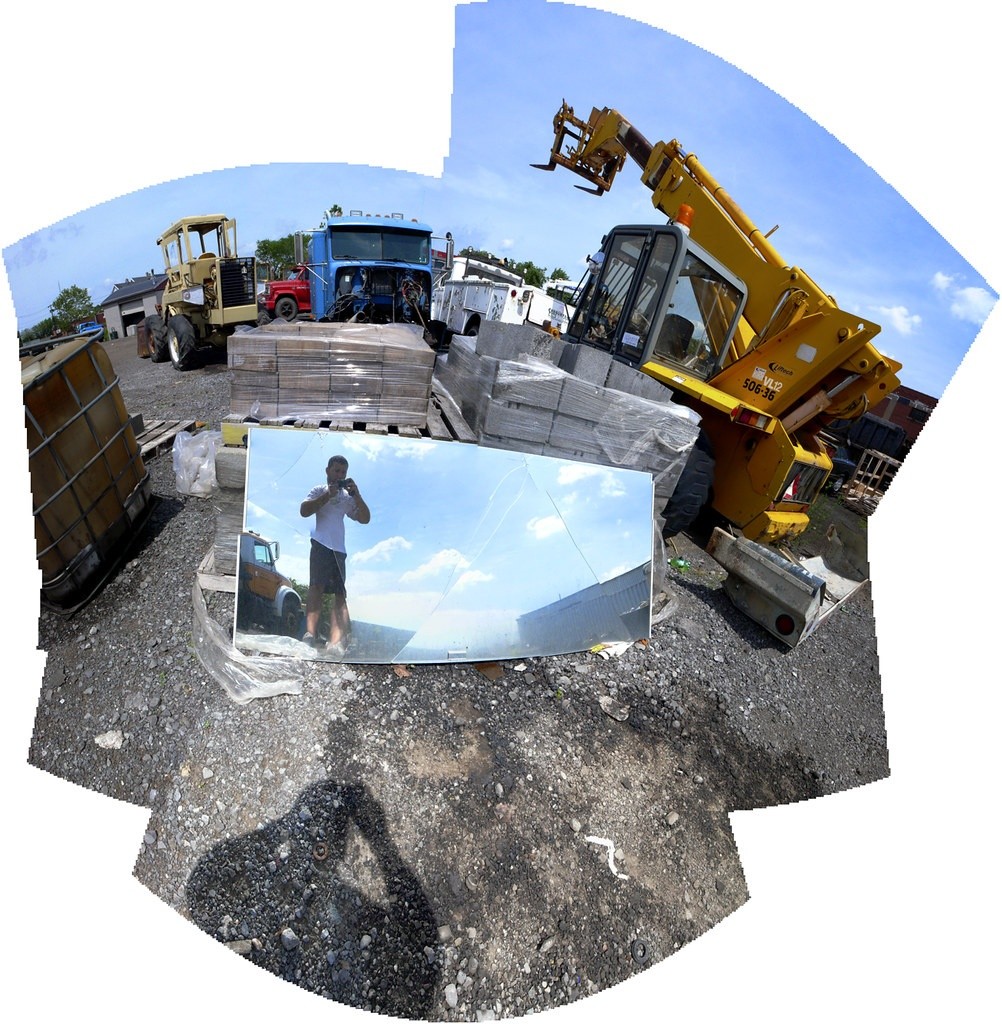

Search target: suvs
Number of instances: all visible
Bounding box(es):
[295,217,455,333]
[258,261,311,324]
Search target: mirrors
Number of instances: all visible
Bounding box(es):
[232,428,654,664]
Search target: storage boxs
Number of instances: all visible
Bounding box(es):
[436,323,702,542]
[226,323,436,427]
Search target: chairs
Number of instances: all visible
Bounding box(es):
[657,315,694,358]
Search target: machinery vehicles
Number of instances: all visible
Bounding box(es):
[136,214,260,373]
[527,102,902,548]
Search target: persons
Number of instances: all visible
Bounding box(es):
[301,454,370,654]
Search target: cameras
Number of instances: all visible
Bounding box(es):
[338,480,348,487]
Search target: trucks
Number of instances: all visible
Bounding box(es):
[239,530,304,635]
[427,245,584,352]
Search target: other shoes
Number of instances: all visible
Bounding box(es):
[302,633,316,648]
[324,641,345,658]
